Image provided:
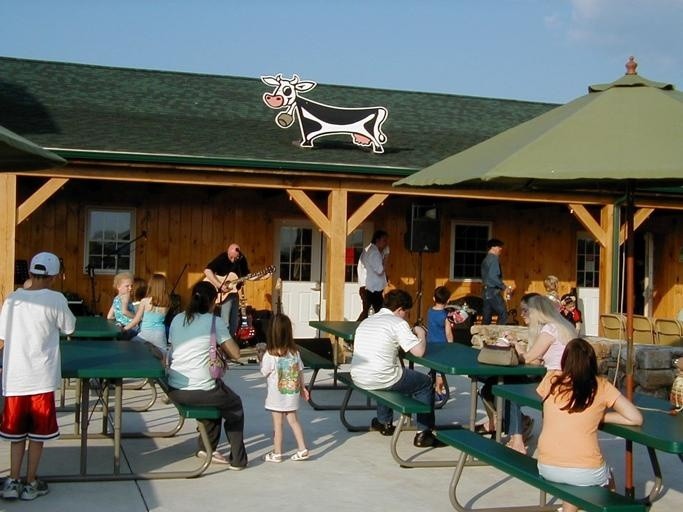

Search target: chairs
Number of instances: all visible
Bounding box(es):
[600,313,682,347]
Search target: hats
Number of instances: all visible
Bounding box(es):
[30,252,60,275]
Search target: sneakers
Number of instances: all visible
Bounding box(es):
[1,477,21,498]
[229,466,244,470]
[198,450,230,464]
[21,479,48,499]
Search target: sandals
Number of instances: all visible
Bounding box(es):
[291,449,309,460]
[262,452,282,462]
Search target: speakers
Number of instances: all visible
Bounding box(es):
[293,338,334,367]
[404,201,440,252]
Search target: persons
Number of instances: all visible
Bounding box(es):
[357,230,388,321]
[426,286,454,400]
[473,276,582,455]
[350,289,447,448]
[481,239,512,325]
[165,281,249,471]
[108,273,138,332]
[121,274,173,362]
[257,314,310,464]
[204,244,250,292]
[1,251,76,501]
[532,338,643,512]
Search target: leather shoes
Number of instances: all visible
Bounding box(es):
[372,417,393,435]
[414,431,448,447]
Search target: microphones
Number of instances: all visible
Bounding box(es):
[236,247,245,258]
[141,230,148,240]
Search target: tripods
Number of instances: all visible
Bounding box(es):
[411,252,428,334]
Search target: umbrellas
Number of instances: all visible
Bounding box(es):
[392,60,682,315]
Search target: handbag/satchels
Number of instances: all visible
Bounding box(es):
[209,347,226,379]
[478,340,519,367]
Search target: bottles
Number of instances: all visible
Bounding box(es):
[367,304,375,318]
[506,285,513,302]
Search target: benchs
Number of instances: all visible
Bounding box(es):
[434,429,634,512]
[337,372,433,466]
[157,377,220,479]
[293,339,339,410]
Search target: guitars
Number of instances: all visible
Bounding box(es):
[202,265,276,304]
[235,285,255,340]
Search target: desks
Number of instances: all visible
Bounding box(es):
[60,340,164,483]
[309,321,362,391]
[60,316,121,337]
[399,343,547,467]
[492,384,683,511]
